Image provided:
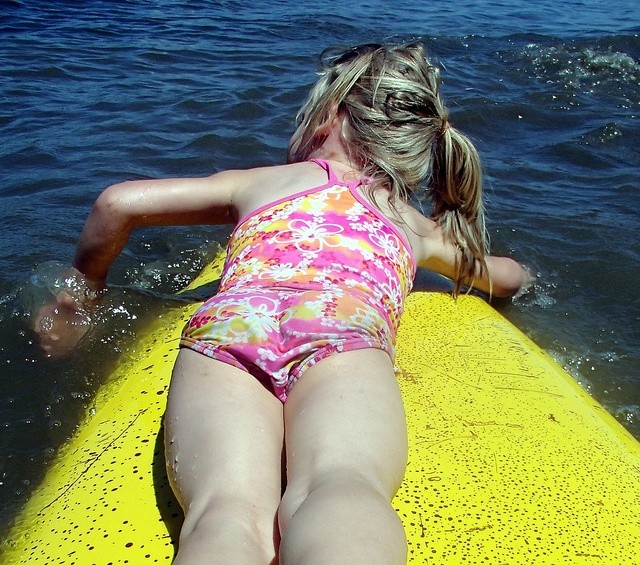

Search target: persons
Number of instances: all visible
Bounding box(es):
[31,41,526,565]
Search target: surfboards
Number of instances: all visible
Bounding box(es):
[0,245,640,565]
